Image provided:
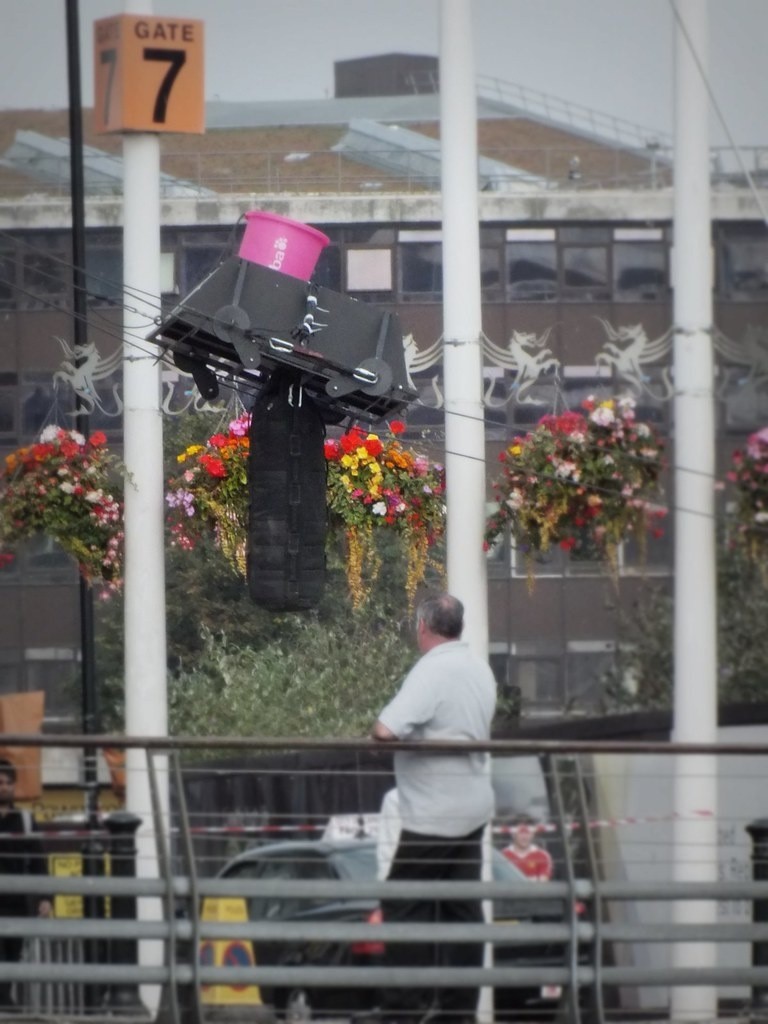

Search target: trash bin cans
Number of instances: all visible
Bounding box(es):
[43,809,144,1006]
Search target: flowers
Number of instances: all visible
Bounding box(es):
[165,412,255,586]
[723,424,767,592]
[0,424,141,601]
[323,419,450,630]
[484,391,669,600]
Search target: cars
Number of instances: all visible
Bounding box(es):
[202,838,589,1023]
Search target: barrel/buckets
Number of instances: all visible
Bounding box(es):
[236,208,330,286]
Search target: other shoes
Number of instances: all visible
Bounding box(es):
[351,1005,464,1024]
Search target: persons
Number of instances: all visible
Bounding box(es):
[0,761,54,1021]
[373,595,496,1023]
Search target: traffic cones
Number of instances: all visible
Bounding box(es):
[195,891,275,1024]
